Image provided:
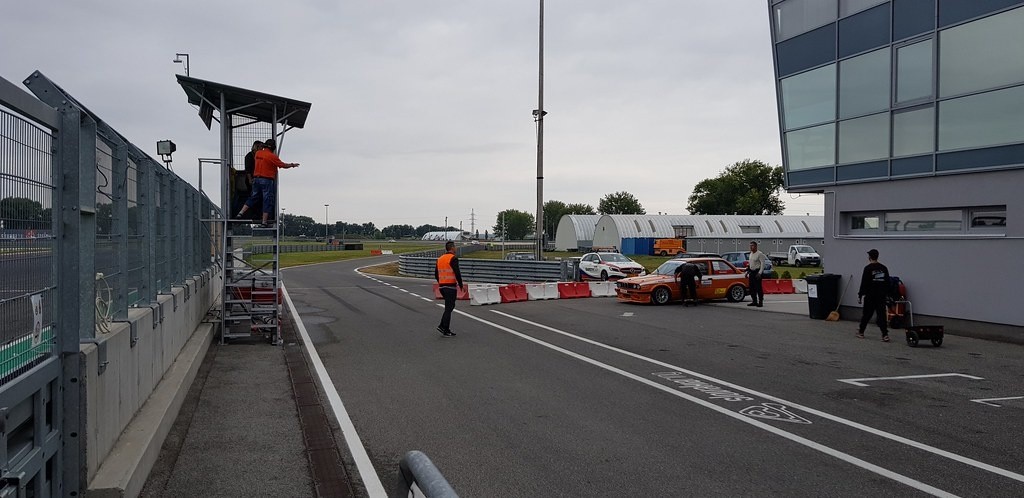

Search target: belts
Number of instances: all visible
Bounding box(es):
[253,175,274,180]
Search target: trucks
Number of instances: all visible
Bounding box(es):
[768,244,821,268]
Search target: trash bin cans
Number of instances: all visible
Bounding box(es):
[806,273,842,320]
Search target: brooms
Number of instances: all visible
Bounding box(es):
[826,275,852,321]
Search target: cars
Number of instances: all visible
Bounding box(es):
[615,252,751,306]
[503,251,547,262]
[388,239,396,243]
[578,252,646,282]
[719,250,775,280]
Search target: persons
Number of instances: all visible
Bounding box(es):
[745,241,766,307]
[236,139,300,227]
[674,263,702,307]
[434,242,466,338]
[854,250,895,342]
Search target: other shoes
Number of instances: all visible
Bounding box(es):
[236,213,243,219]
[446,330,456,336]
[682,301,688,306]
[260,223,273,228]
[856,333,864,338]
[747,302,758,306]
[261,215,272,220]
[437,325,451,337]
[881,336,889,342]
[756,302,763,307]
[693,300,697,306]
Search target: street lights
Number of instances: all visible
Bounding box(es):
[325,205,329,236]
[282,208,285,237]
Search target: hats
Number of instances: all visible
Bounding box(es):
[867,249,879,256]
[262,139,276,150]
